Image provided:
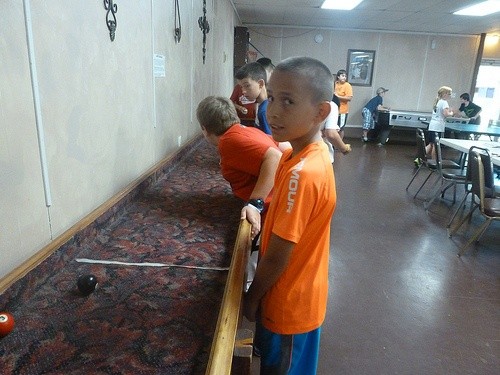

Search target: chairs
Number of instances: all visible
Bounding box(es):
[426,132,474,223]
[447,145,500,243]
[448,151,500,258]
[406,128,461,204]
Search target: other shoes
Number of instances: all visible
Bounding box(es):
[414,157,420,168]
[360,136,369,143]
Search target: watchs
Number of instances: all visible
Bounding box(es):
[246,198,265,213]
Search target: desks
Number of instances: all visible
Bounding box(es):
[421,121,500,165]
[377,108,470,146]
[439,135,500,167]
[0,117,256,375]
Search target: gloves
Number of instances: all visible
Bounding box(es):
[342,144,352,154]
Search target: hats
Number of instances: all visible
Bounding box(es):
[377,86,389,93]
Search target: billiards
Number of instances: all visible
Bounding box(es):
[0,313,15,334]
[77,274,99,294]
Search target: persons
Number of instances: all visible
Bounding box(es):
[196,96,292,358]
[456,93,482,125]
[415,86,455,168]
[228,84,256,126]
[256,57,274,83]
[334,70,353,141]
[243,55,337,375]
[235,62,273,136]
[320,101,352,166]
[361,87,389,143]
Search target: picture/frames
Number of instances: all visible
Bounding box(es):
[346,49,376,87]
